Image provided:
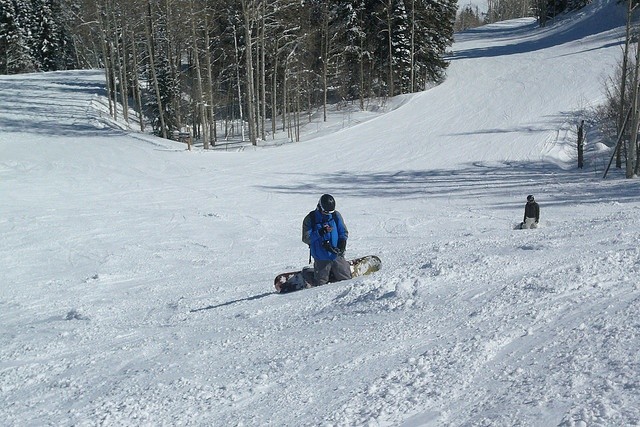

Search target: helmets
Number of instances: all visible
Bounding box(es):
[318,194,336,214]
[527,194,536,203]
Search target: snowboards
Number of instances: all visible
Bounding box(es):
[274,255,383,295]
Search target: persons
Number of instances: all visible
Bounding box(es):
[520,195,540,229]
[302,193,351,286]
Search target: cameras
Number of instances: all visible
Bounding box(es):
[321,239,337,257]
